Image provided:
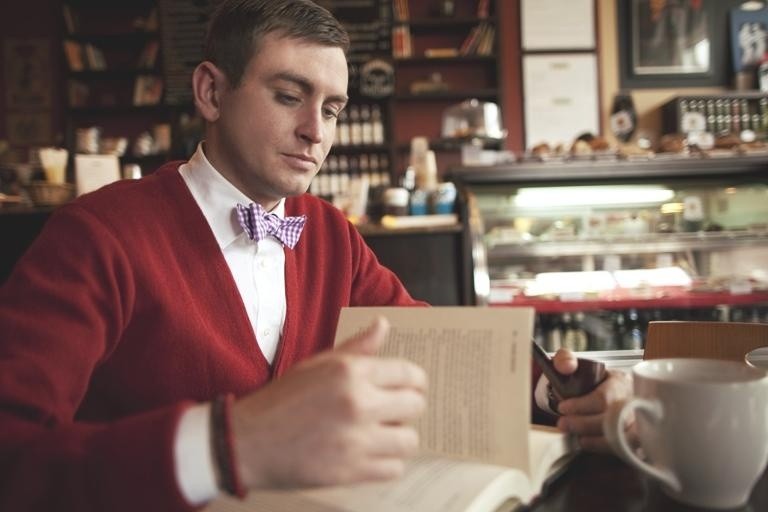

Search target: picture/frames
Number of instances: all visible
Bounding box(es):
[628,2,713,77]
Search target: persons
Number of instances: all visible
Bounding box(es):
[0,1,633,512]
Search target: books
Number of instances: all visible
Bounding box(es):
[200,306,586,512]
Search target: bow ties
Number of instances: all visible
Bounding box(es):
[236,201,307,248]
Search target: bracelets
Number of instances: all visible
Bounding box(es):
[211,392,247,498]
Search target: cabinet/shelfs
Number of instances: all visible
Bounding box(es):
[387,1,522,187]
[450,152,766,316]
[63,1,208,184]
[303,13,399,215]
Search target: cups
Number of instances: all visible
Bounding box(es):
[600,356,768,509]
[36,147,70,186]
